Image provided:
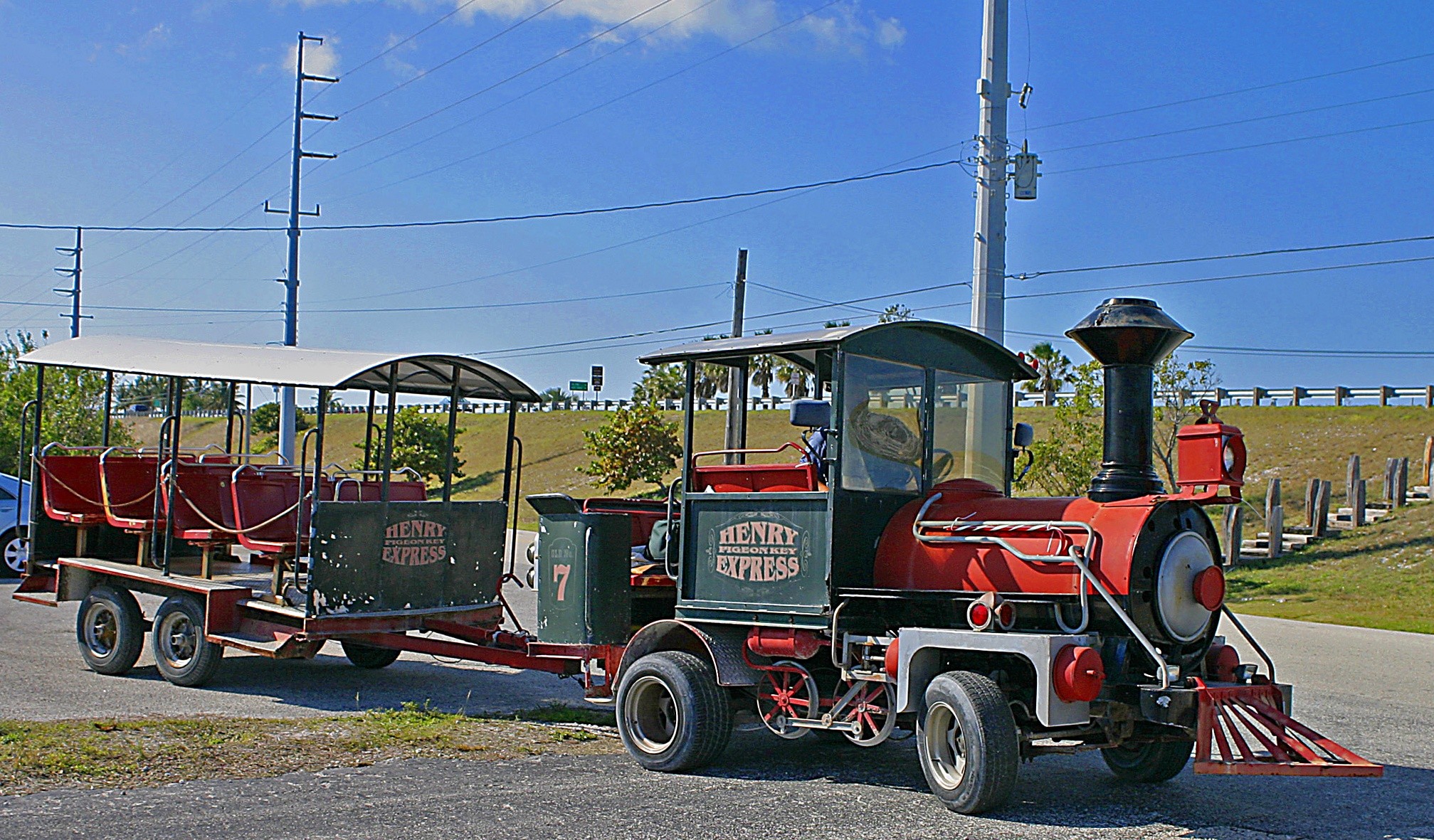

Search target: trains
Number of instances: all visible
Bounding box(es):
[15,288,1389,815]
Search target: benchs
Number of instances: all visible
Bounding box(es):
[160,458,253,579]
[261,463,352,476]
[332,465,428,503]
[139,447,196,461]
[199,450,291,474]
[40,441,139,557]
[162,444,231,464]
[691,443,819,493]
[582,497,680,587]
[231,464,333,607]
[98,447,199,568]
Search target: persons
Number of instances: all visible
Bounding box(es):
[799,379,922,491]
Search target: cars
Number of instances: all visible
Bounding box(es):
[0,471,34,580]
